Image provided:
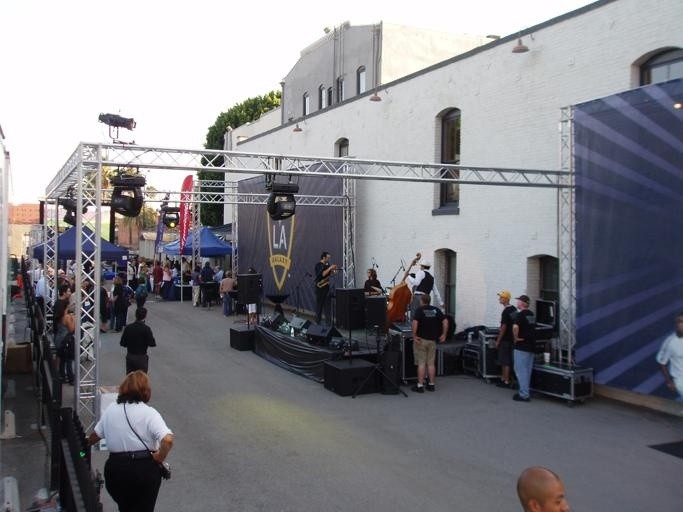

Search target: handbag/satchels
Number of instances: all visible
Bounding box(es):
[159,462,170,480]
[61,332,76,360]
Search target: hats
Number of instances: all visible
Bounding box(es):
[419,260,431,267]
[496,292,510,300]
[514,295,529,304]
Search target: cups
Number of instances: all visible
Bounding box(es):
[543,353,550,362]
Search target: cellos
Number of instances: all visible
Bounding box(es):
[387,253,421,322]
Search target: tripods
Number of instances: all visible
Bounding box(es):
[353,336,408,397]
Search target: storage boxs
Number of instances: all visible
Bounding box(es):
[478,329,507,380]
[93,385,123,451]
[387,328,439,383]
[529,360,594,404]
[533,297,560,341]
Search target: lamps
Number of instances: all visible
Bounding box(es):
[108,170,148,217]
[60,185,88,225]
[161,203,182,229]
[95,111,140,144]
[510,26,537,55]
[263,180,299,221]
[368,81,390,103]
[292,113,306,133]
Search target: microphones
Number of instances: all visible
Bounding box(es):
[401,260,405,271]
[373,257,378,268]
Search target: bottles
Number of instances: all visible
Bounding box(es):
[467,334,472,343]
[290,327,294,337]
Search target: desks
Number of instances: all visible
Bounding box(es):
[82,266,224,324]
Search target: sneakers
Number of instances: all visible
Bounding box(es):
[495,381,510,388]
[425,385,433,391]
[411,386,423,393]
[512,393,530,402]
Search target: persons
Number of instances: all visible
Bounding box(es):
[412,294,450,394]
[363,269,384,297]
[26,256,258,386]
[494,291,518,389]
[516,465,570,512]
[512,295,537,402]
[86,371,173,512]
[314,252,337,327]
[402,260,434,332]
[655,310,683,406]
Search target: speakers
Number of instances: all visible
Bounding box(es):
[289,317,311,332]
[236,274,259,303]
[307,325,342,346]
[337,288,365,330]
[367,296,386,336]
